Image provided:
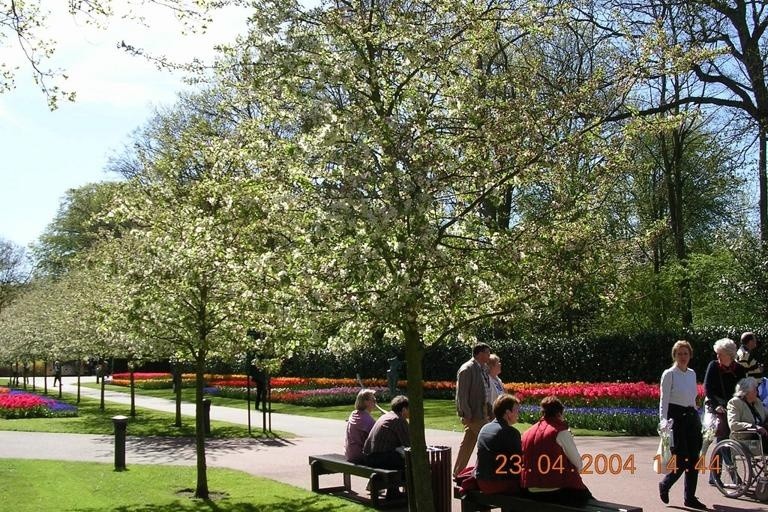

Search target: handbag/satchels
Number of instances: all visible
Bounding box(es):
[701,411,731,439]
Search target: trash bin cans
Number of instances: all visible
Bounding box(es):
[403,446,451,512]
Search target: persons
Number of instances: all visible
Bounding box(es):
[344,389,384,491]
[251,368,268,411]
[361,395,411,500]
[23,360,29,384]
[53,359,64,387]
[658,331,768,510]
[453,342,593,512]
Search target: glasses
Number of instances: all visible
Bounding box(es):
[676,351,689,357]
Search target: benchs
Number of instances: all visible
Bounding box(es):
[450,486,642,512]
[307,452,408,510]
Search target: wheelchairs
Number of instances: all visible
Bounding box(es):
[710,407,768,499]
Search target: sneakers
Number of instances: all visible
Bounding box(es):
[657,481,670,505]
[682,495,707,512]
